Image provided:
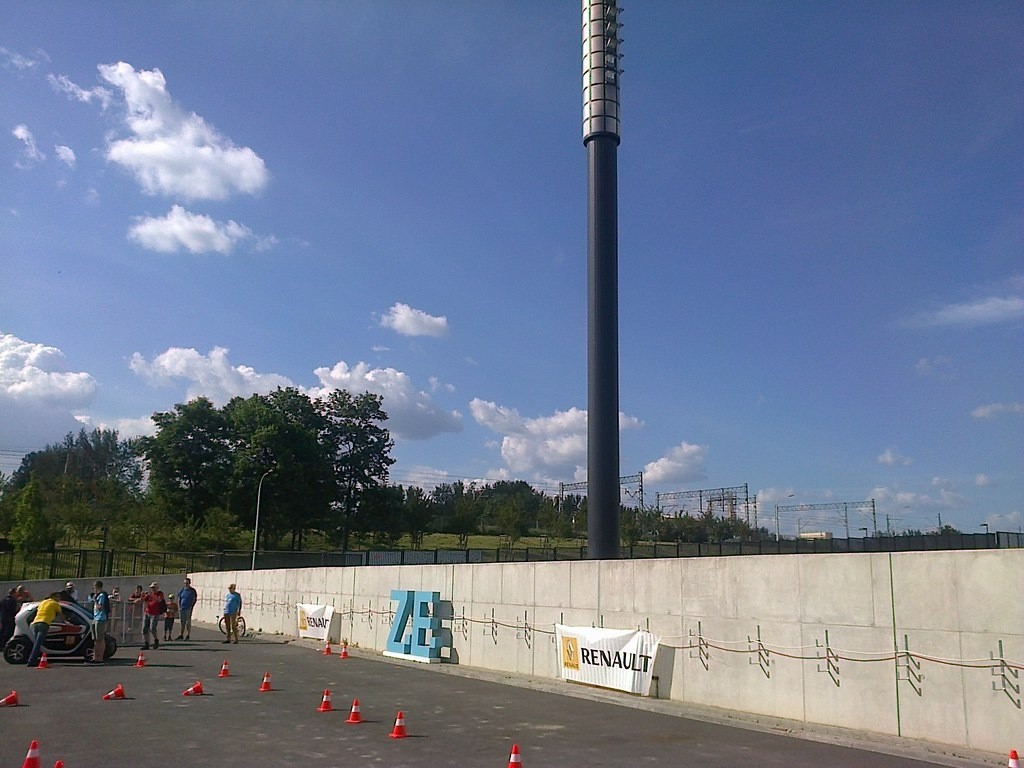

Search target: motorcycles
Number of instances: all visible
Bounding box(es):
[4,601,117,663]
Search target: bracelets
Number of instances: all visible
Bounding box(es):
[94,600,97,604]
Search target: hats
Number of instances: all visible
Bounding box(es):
[228,584,236,589]
[168,594,175,599]
[149,582,159,588]
[66,582,75,588]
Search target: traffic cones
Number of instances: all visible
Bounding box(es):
[1008,749,1024,768]
[322,641,334,655]
[102,685,126,700]
[183,681,204,696]
[258,672,274,691]
[507,744,524,768]
[317,689,336,712]
[388,711,412,739]
[338,645,350,659]
[219,661,229,678]
[134,652,145,668]
[345,699,367,723]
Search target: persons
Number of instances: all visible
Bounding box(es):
[127,585,143,632]
[140,582,164,650]
[66,582,79,603]
[175,579,197,641]
[26,593,69,667]
[0,585,36,652]
[163,594,178,641]
[221,584,242,644]
[84,581,109,666]
[108,586,123,603]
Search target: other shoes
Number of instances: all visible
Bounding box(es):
[174,635,184,641]
[163,635,172,641]
[222,640,231,644]
[84,660,105,665]
[184,635,190,641]
[233,641,238,644]
[27,663,38,667]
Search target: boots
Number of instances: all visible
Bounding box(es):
[152,639,159,649]
[140,642,150,650]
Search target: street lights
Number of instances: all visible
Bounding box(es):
[979,522,989,549]
[775,494,795,532]
[250,469,276,571]
[859,528,868,536]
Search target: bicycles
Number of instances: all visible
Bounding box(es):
[219,607,246,637]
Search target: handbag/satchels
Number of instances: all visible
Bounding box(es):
[157,591,167,614]
